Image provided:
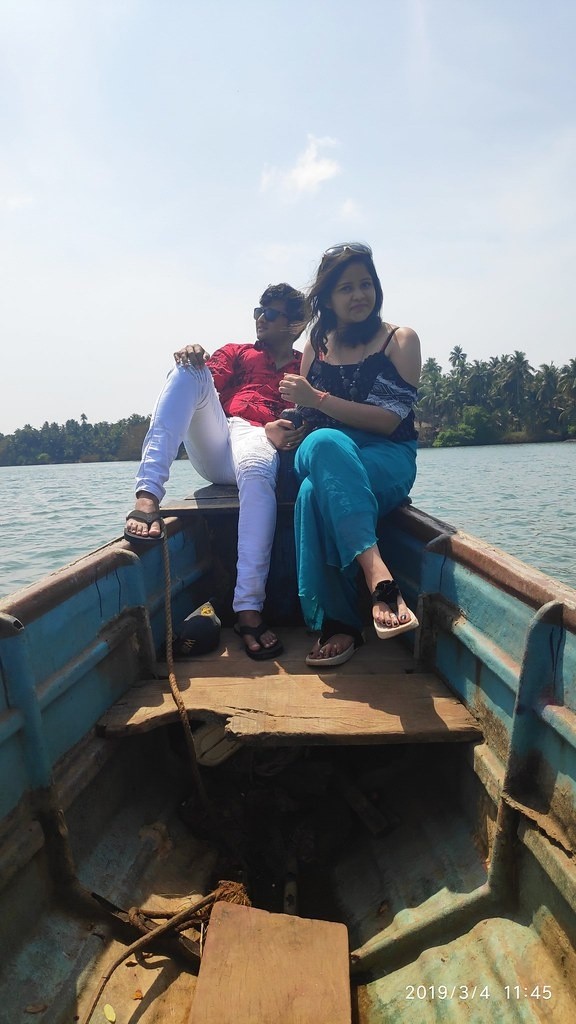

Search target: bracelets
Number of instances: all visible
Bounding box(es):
[316,387,331,409]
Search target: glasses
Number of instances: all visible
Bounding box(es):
[323,242,372,260]
[253,306,292,323]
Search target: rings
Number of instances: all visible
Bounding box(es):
[287,442,291,448]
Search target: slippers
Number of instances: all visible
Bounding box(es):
[304,617,367,668]
[125,508,167,547]
[370,578,421,639]
[233,618,285,662]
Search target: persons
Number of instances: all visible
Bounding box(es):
[125,284,312,661]
[264,242,421,670]
